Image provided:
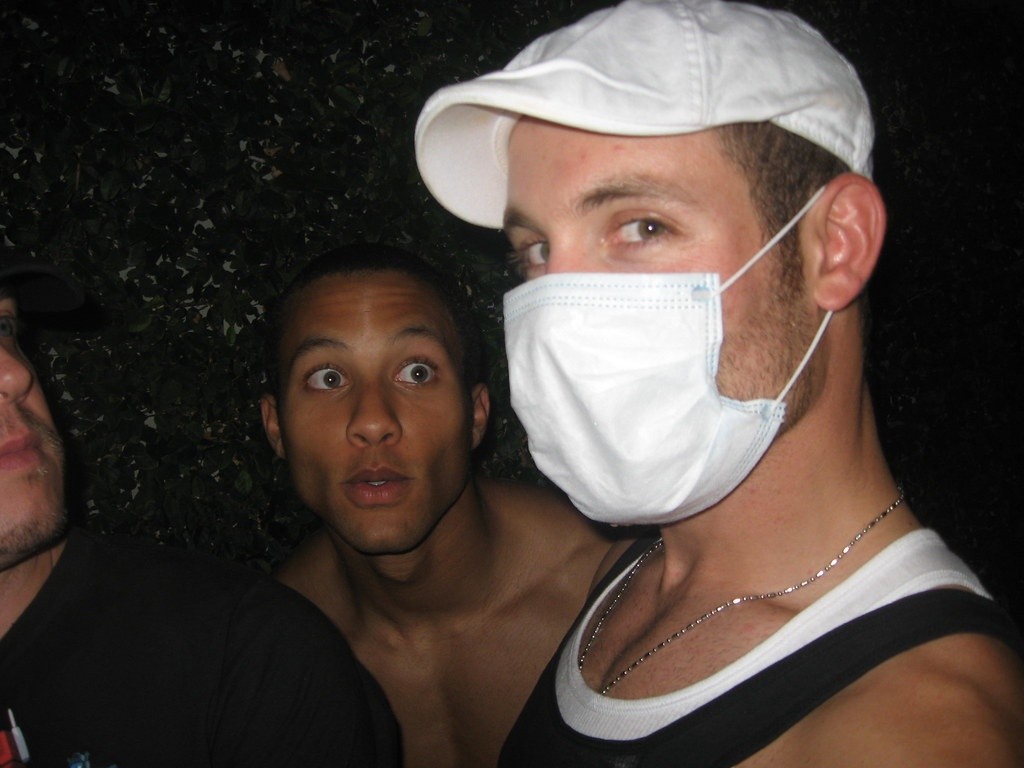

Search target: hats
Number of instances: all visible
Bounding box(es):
[414,0,875,231]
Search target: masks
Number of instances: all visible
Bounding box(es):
[500,184,839,525]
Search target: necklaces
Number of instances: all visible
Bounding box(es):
[576,487,907,698]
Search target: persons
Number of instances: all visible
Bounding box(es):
[0,244,615,767]
[413,0,1024,768]
